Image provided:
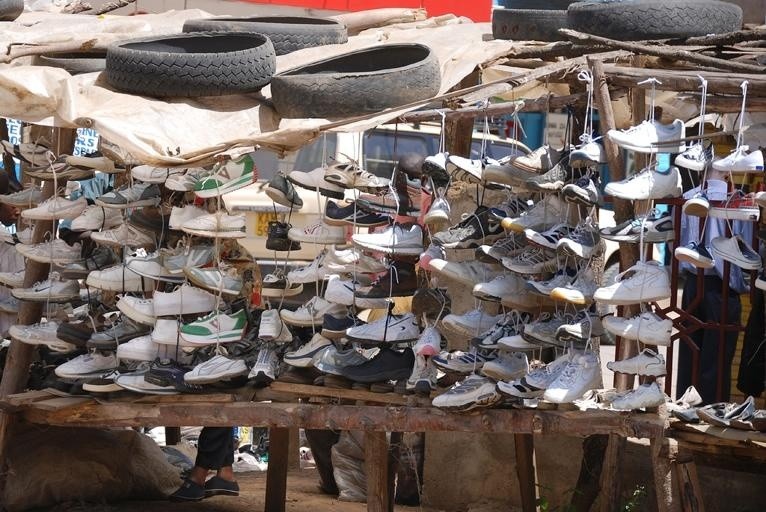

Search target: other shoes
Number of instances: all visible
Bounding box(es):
[665,386,766,429]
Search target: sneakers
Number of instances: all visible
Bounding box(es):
[604,155,684,200]
[675,76,765,271]
[598,208,675,244]
[602,310,673,349]
[612,382,666,410]
[607,78,688,155]
[1,139,607,414]
[594,259,673,305]
[206,473,241,496]
[606,347,668,379]
[166,478,206,502]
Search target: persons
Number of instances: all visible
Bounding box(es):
[397,152,426,179]
[667,176,753,404]
[172,426,240,502]
[59,169,116,239]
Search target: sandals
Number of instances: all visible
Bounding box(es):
[671,395,766,429]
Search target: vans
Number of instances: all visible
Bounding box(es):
[243,123,533,181]
